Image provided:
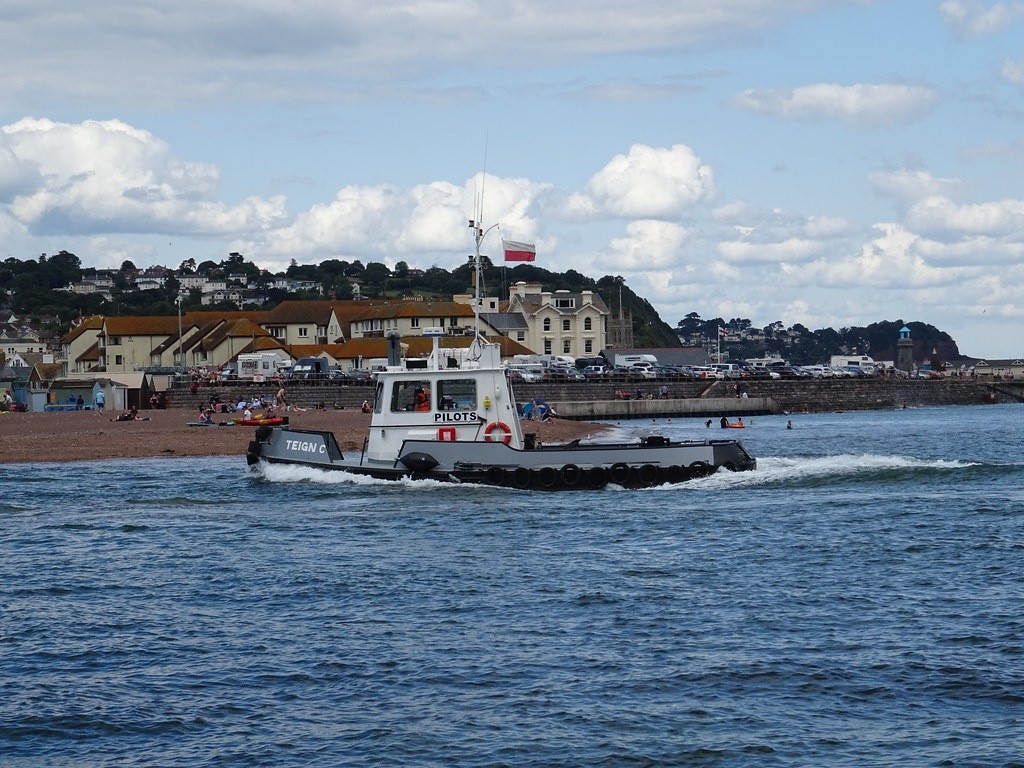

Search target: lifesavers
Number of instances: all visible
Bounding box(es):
[485,466,506,486]
[610,462,631,485]
[587,466,609,489]
[664,465,685,485]
[638,464,659,487]
[512,467,531,489]
[688,460,706,479]
[559,464,581,486]
[539,467,558,488]
[484,422,512,446]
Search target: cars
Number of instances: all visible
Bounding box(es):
[328,369,376,386]
[505,352,945,383]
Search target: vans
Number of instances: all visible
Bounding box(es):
[288,358,330,385]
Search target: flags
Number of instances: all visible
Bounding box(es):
[503,240,535,261]
[719,327,729,336]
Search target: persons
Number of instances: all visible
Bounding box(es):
[733,382,749,399]
[77,394,84,405]
[635,383,670,400]
[110,405,151,421]
[873,364,921,380]
[787,421,792,429]
[703,416,742,429]
[607,368,615,384]
[197,387,305,421]
[528,400,559,426]
[361,400,373,413]
[188,364,224,393]
[3,393,12,409]
[950,366,1015,381]
[149,393,165,410]
[69,394,76,403]
[95,390,105,416]
[413,387,429,412]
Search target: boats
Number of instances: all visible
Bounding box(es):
[231,415,290,428]
[186,422,234,426]
[245,216,758,491]
